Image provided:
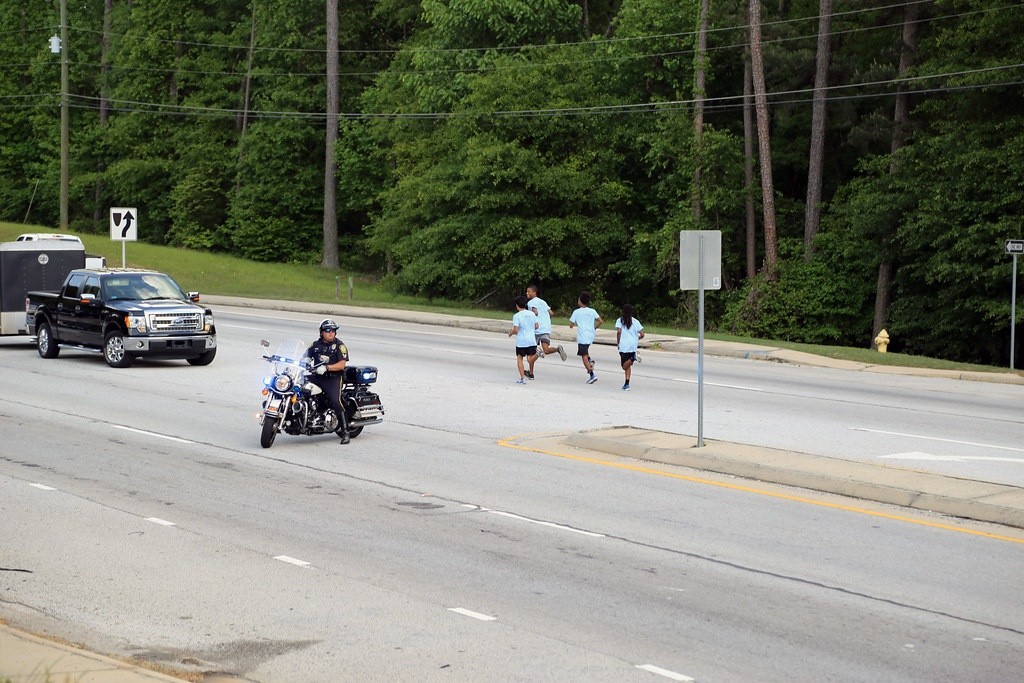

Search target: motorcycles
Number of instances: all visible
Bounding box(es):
[258,338,385,449]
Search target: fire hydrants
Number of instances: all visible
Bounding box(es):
[874,328,891,354]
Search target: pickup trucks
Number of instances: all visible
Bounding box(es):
[26,268,217,370]
[14,233,106,268]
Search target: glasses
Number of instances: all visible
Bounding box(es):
[323,328,336,333]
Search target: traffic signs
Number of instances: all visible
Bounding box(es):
[1003,238,1024,255]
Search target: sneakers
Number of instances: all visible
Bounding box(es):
[536,347,545,359]
[587,374,597,384]
[524,370,535,380]
[634,351,642,363]
[516,379,527,385]
[557,345,567,361]
[587,359,595,374]
[622,385,630,391]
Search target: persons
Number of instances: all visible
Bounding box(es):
[300,320,351,445]
[526,285,567,361]
[508,297,545,385]
[570,293,604,384]
[615,303,645,391]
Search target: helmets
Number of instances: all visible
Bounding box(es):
[320,319,339,330]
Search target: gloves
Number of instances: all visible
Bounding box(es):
[304,357,312,364]
[317,365,327,375]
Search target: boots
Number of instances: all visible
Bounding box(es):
[337,411,351,444]
